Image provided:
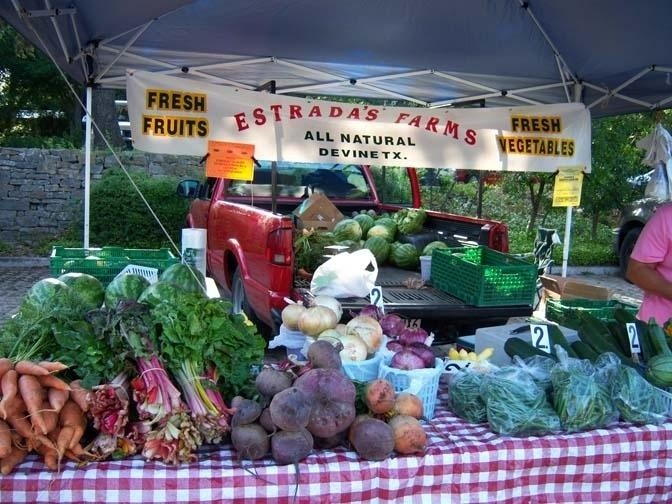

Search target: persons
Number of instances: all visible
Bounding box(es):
[623,200,672,328]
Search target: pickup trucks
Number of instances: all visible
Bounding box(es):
[176,155,534,353]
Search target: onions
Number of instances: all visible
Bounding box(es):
[281,296,434,370]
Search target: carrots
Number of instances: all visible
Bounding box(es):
[0,358,101,476]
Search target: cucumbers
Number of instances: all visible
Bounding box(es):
[505,307,672,387]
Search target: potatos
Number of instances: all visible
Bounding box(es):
[228,341,425,465]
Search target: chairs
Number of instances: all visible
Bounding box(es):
[531,225,561,312]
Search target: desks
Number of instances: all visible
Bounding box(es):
[0,380,672,504]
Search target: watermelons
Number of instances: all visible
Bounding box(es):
[20,263,207,323]
[332,214,449,270]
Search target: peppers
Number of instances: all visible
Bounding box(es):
[448,353,666,435]
